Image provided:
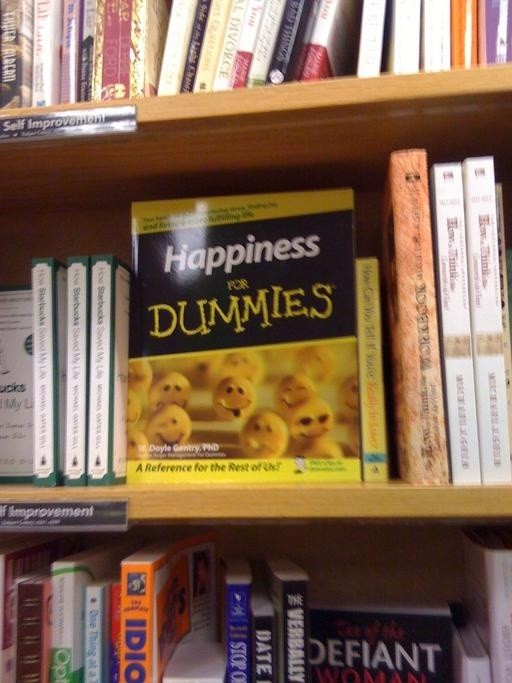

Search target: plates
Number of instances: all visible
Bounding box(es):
[0,64,512,527]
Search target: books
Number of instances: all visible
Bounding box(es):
[0,0,510,106]
[124,189,388,486]
[387,149,511,488]
[2,253,126,488]
[2,537,511,683]
